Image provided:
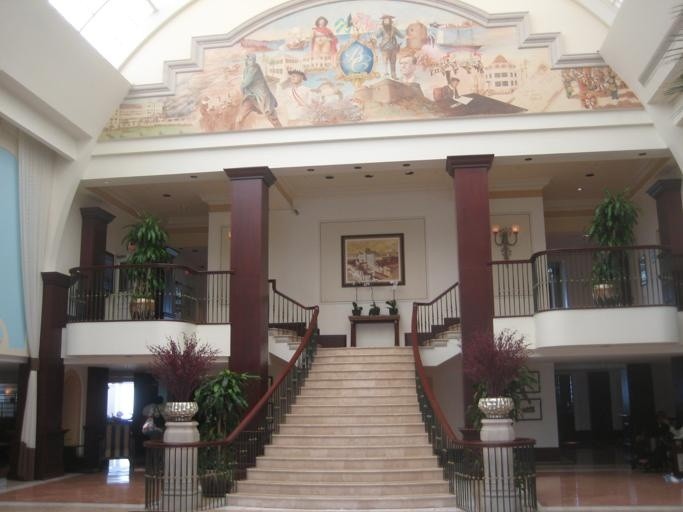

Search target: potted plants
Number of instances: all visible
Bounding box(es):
[196,366,262,498]
[581,185,643,309]
[352,301,363,316]
[386,299,398,316]
[369,302,380,316]
[120,206,174,321]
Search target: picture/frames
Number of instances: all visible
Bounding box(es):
[639,254,648,287]
[517,371,541,394]
[103,251,114,293]
[516,398,543,421]
[341,232,406,287]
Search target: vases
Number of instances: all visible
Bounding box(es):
[162,400,204,422]
[479,393,516,417]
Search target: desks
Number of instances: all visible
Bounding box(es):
[348,315,400,347]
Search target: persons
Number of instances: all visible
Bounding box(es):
[231,54,280,132]
[309,16,338,57]
[286,67,311,111]
[433,77,461,104]
[374,15,405,81]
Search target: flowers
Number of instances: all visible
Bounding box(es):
[455,325,529,396]
[147,332,218,400]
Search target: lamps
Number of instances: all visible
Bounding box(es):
[491,223,520,259]
